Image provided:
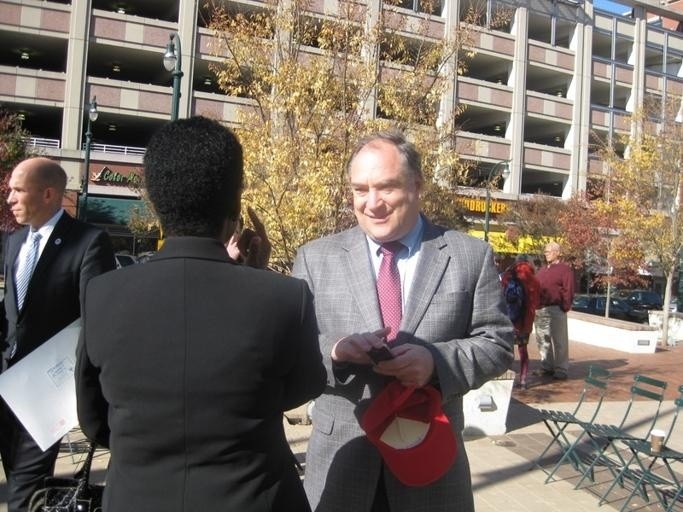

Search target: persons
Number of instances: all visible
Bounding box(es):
[500,241,576,389]
[76,115,327,512]
[2,157,118,511]
[293,134,517,512]
[223,217,244,263]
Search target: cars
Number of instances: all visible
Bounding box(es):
[571,290,683,325]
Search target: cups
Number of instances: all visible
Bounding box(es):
[650,429,665,453]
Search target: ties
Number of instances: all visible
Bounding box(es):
[15,234,43,313]
[374,242,404,341]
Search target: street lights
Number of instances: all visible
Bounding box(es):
[163,32,184,120]
[484,159,510,242]
[78,95,99,222]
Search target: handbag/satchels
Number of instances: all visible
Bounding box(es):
[26,440,105,512]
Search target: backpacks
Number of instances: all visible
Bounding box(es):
[505,279,525,323]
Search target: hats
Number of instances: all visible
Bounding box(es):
[359,378,459,487]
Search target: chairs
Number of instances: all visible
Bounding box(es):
[527,365,682,512]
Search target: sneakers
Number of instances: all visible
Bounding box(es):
[532,367,567,380]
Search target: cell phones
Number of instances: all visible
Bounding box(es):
[366,344,395,368]
